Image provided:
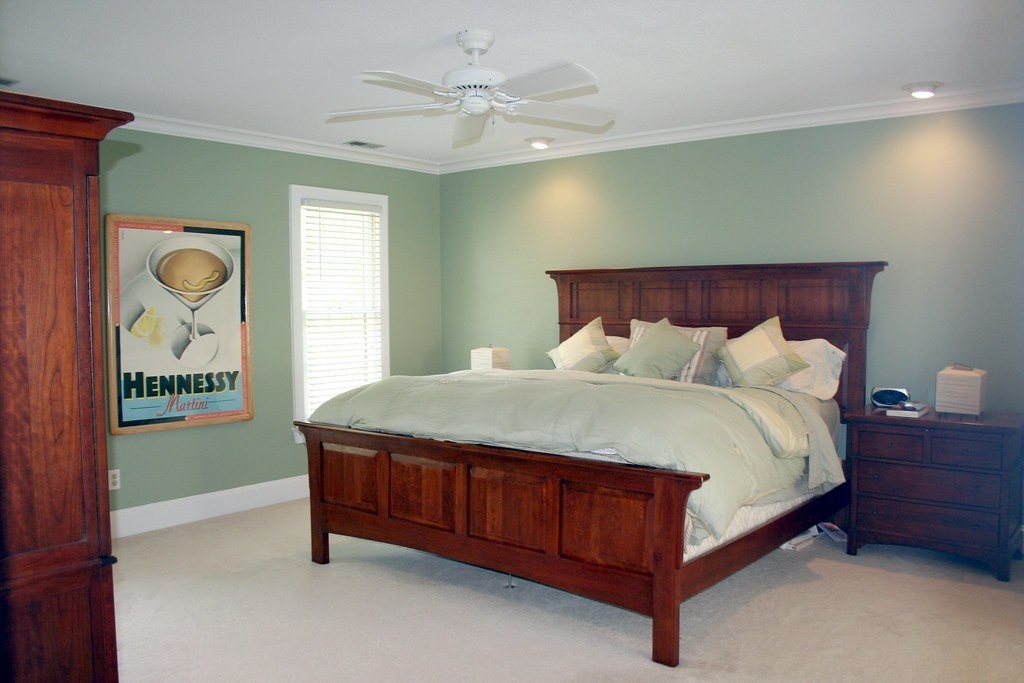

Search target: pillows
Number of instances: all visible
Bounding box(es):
[545,316,620,373]
[717,338,847,401]
[629,319,728,385]
[552,336,630,374]
[612,318,701,379]
[713,316,811,388]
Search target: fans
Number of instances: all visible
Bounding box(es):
[325,30,614,146]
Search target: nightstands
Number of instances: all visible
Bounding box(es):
[844,407,1024,582]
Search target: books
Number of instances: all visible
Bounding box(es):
[886,402,929,419]
[779,523,848,551]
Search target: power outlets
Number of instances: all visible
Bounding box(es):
[108,469,121,491]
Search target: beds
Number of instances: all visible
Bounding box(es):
[294,261,890,668]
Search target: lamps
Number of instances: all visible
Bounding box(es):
[524,137,553,150]
[902,81,945,101]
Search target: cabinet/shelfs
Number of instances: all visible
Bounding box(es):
[1,92,135,683]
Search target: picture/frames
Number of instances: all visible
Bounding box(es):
[106,214,254,435]
